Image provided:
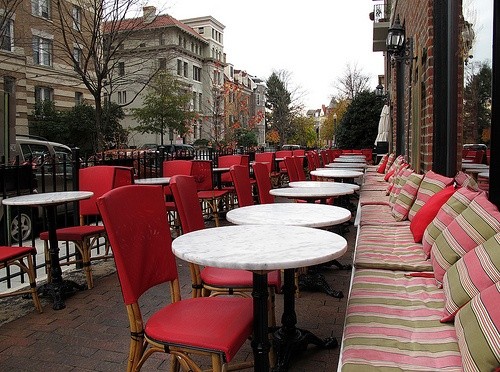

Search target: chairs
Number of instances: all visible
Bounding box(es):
[0,146,373,372]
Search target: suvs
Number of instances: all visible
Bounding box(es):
[0,133,93,244]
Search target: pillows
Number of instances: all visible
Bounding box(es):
[376,148,500,372]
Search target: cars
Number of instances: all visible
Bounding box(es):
[89,148,160,180]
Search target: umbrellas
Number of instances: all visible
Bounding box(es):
[374,105,389,147]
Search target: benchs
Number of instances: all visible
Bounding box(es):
[335,164,500,372]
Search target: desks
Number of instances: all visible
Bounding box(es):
[287,180,360,204]
[2,190,94,311]
[224,201,352,368]
[268,186,354,298]
[341,152,363,155]
[274,157,285,172]
[339,155,366,158]
[134,176,171,187]
[325,163,365,168]
[333,158,366,162]
[309,169,363,184]
[212,167,231,189]
[170,224,348,372]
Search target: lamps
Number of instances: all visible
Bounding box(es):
[385,13,417,69]
[375,83,390,106]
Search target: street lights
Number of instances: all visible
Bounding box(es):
[333,112,337,148]
